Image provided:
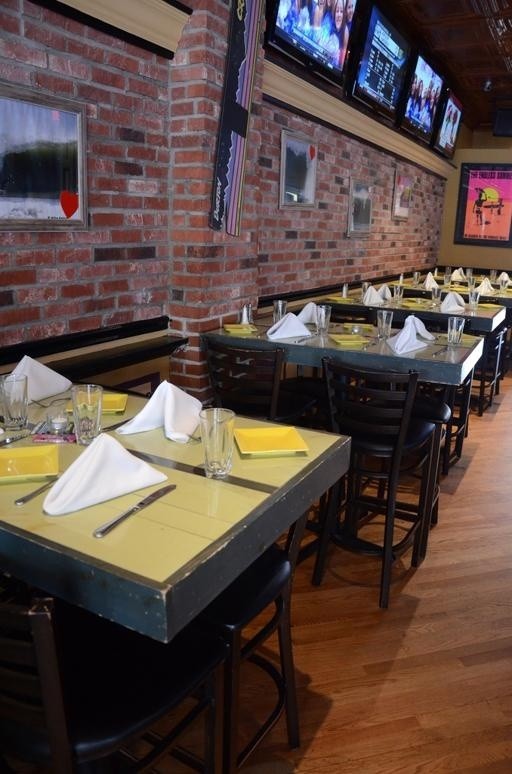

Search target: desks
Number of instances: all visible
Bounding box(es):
[0,375,354,769]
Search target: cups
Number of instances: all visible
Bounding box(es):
[393,285,404,301]
[448,317,466,343]
[70,385,103,446]
[313,305,331,335]
[431,287,442,307]
[444,266,451,279]
[411,271,420,288]
[44,408,68,432]
[500,278,508,293]
[362,282,372,302]
[377,310,393,340]
[465,268,474,283]
[0,374,28,428]
[443,275,451,289]
[273,300,288,325]
[199,408,235,480]
[467,277,476,292]
[490,269,498,283]
[469,291,479,309]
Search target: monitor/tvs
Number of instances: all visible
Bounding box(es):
[432,86,465,160]
[351,0,414,125]
[397,50,444,145]
[265,0,362,91]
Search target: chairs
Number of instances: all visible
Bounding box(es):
[3,538,302,767]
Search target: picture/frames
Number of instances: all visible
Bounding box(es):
[0,78,89,233]
[278,130,318,211]
[391,167,413,222]
[346,177,374,238]
[453,162,512,248]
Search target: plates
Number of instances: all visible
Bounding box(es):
[449,287,470,292]
[326,334,369,348]
[400,302,425,309]
[0,443,60,487]
[404,298,430,303]
[479,303,504,310]
[389,282,412,287]
[230,425,311,460]
[344,323,373,334]
[442,285,465,289]
[222,324,256,336]
[65,392,129,414]
[496,289,512,295]
[327,297,355,304]
[439,333,476,342]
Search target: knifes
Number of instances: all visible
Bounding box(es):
[294,334,317,344]
[93,484,176,538]
[432,345,449,357]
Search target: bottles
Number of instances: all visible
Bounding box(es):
[249,303,254,323]
[434,268,439,276]
[241,304,249,324]
[342,283,349,298]
[400,273,404,284]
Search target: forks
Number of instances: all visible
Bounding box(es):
[31,397,69,408]
[362,338,379,350]
[186,423,218,443]
[0,421,45,446]
